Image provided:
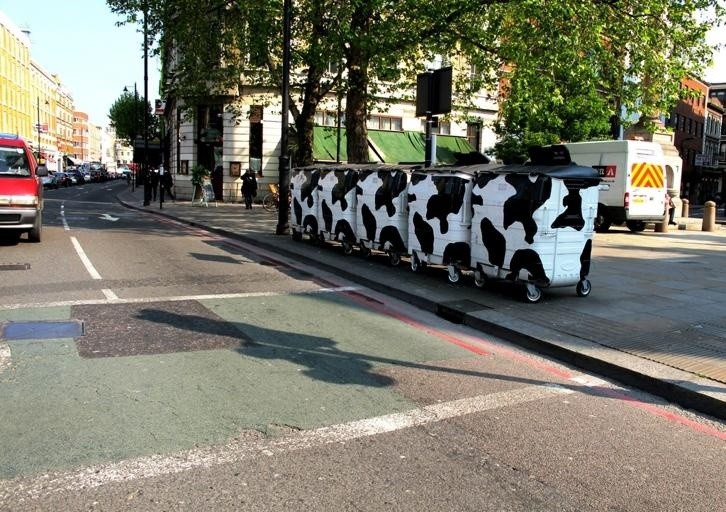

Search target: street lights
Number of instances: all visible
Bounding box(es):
[123,82,137,137]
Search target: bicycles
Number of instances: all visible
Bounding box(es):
[263,183,291,212]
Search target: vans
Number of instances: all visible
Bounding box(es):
[523,140,667,234]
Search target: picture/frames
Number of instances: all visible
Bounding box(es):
[229,161,241,177]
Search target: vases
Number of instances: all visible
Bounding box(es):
[180,159,189,175]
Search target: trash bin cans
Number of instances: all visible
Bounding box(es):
[469,162,602,302]
[290,164,326,243]
[355,162,423,267]
[316,163,370,256]
[405,164,492,283]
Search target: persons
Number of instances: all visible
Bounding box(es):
[162,170,174,202]
[146,168,160,201]
[240,168,258,209]
[668,193,678,226]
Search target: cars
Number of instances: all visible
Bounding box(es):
[0,133,131,242]
